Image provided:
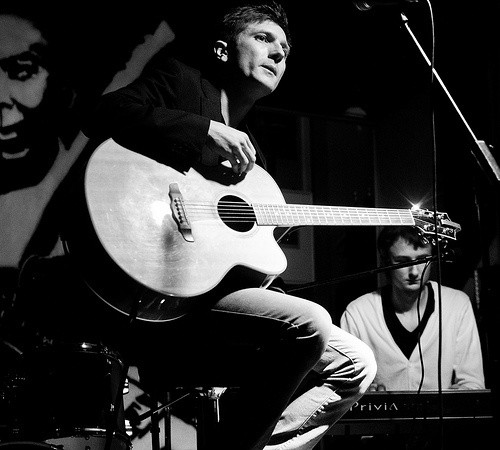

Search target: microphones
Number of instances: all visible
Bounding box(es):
[352,0,421,11]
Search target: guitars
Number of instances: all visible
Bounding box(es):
[59,134,464,321]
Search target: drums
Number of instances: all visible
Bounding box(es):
[21,333,135,449]
[1,336,48,449]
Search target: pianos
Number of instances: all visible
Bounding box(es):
[337,387,498,424]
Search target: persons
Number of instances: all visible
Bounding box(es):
[1,0,79,267]
[78,1,377,450]
[339,224,485,391]
